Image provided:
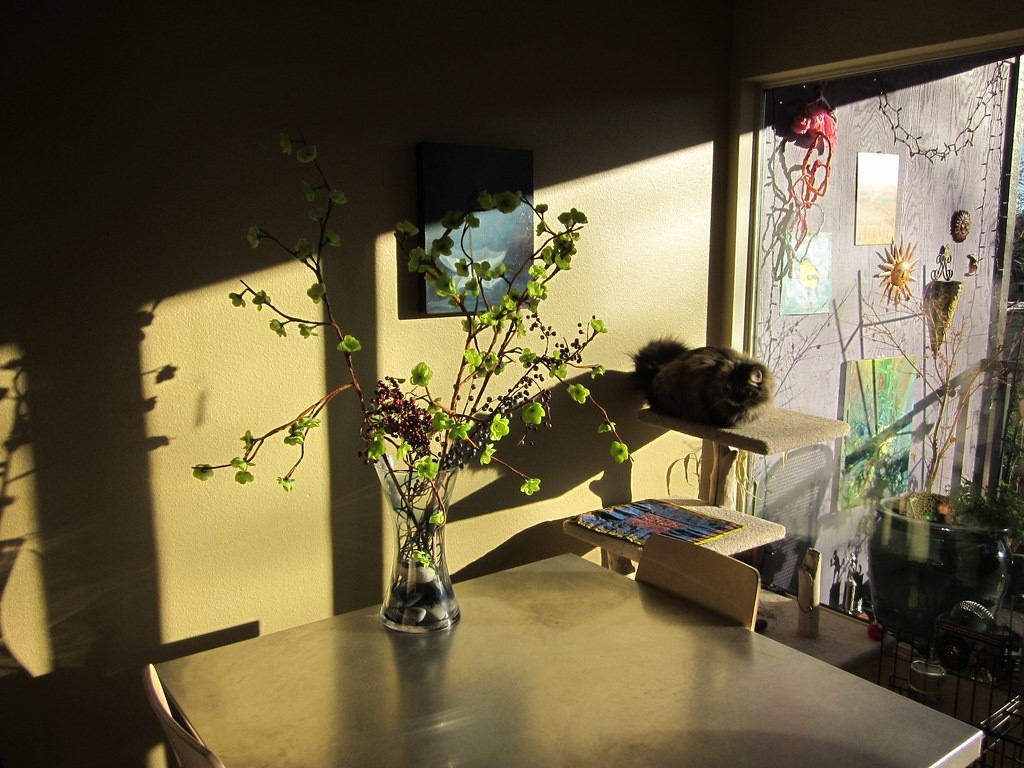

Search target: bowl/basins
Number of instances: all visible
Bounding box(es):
[438,245,508,275]
[451,275,508,289]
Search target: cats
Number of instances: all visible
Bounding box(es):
[633,335,776,429]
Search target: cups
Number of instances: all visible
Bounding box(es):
[907,659,947,709]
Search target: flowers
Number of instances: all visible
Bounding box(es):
[192,126,632,571]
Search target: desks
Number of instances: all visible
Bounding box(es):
[151,553,985,768]
[754,583,897,672]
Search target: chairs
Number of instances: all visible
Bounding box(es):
[142,663,224,768]
[634,533,761,633]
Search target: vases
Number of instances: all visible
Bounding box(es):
[373,453,461,635]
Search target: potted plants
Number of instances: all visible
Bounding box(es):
[855,279,1024,648]
